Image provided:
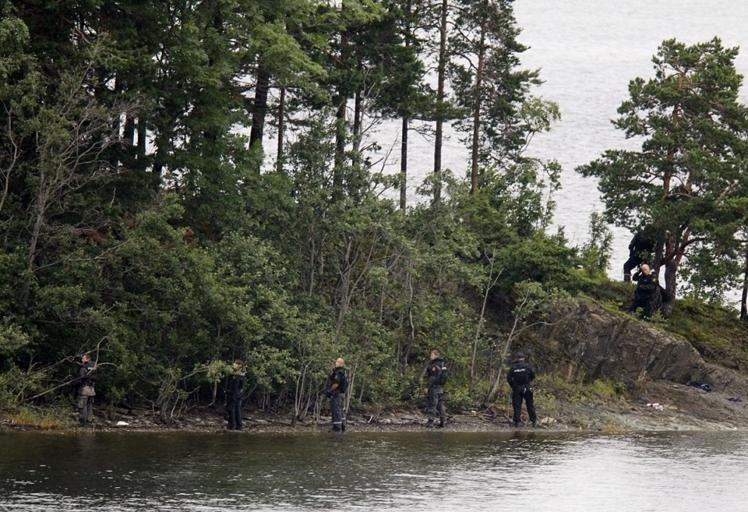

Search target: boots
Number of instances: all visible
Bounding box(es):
[332,422,345,433]
[437,420,445,427]
[424,420,433,427]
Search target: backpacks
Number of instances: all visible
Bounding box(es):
[432,363,448,385]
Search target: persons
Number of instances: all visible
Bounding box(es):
[623,228,652,286]
[325,357,349,434]
[222,358,249,432]
[506,352,539,428]
[75,353,98,427]
[629,264,658,321]
[423,349,447,429]
